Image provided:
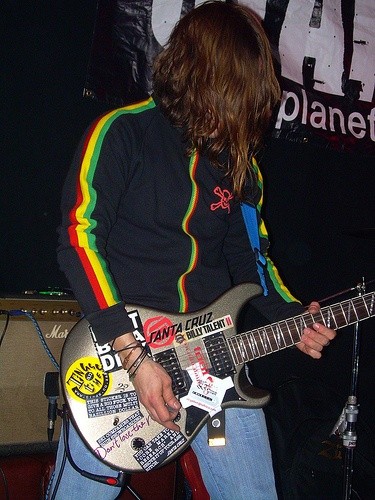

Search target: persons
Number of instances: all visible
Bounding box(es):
[46,0,336,499]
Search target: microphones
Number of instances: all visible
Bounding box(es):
[43,371,60,441]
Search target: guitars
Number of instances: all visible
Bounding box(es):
[60,284,375,473]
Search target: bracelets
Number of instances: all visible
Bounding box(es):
[110,338,148,378]
